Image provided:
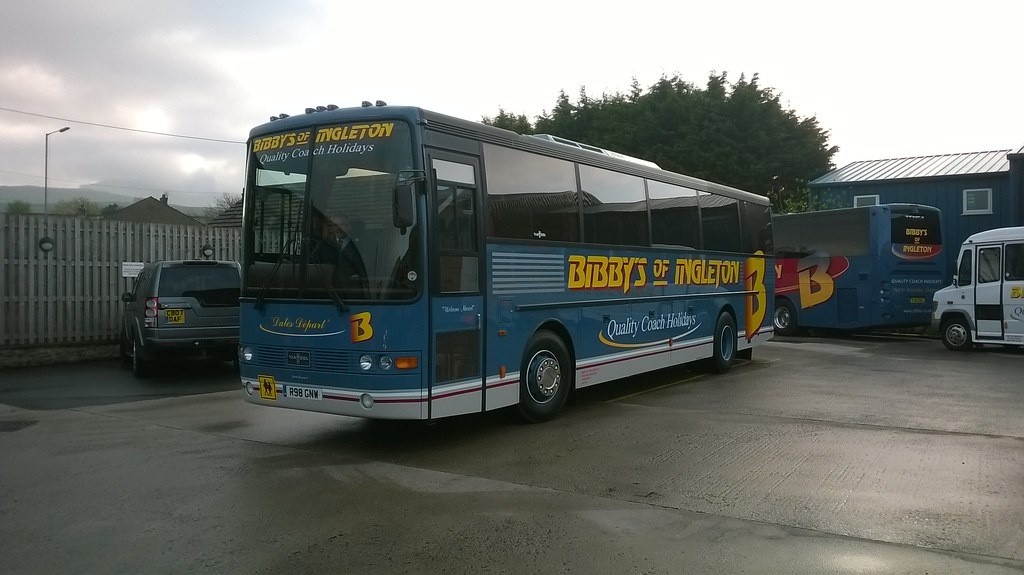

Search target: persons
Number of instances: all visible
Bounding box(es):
[312,197,383,290]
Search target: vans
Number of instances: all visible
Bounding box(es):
[931,225,1024,351]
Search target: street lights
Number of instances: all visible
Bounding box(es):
[44,126,70,214]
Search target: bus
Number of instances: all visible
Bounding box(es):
[772,202,948,337]
[237,99,776,425]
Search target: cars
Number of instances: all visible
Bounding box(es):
[120,258,243,376]
